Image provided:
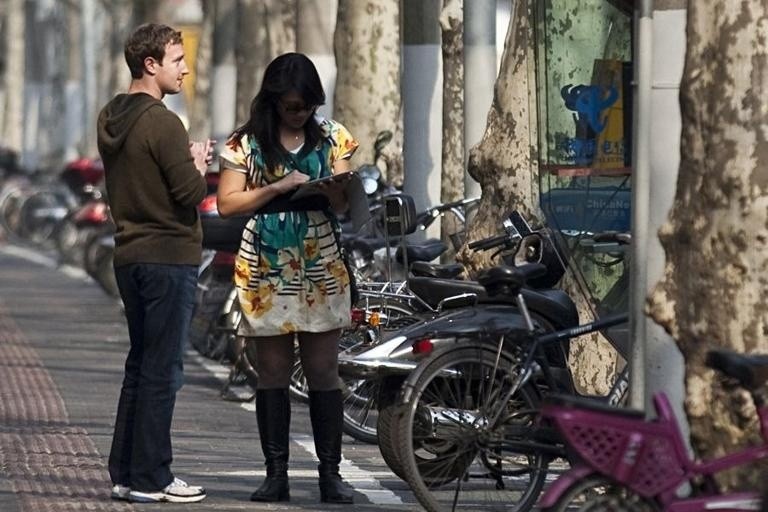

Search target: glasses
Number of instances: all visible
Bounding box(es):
[279,99,320,116]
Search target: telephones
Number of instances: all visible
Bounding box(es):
[574,60,631,168]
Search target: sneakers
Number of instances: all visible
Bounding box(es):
[109,476,207,505]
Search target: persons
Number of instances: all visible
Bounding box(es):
[213,51,358,504]
[94,23,219,504]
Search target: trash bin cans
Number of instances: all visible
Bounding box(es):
[541,189,632,309]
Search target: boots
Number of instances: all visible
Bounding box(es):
[306,387,356,506]
[248,387,292,504]
